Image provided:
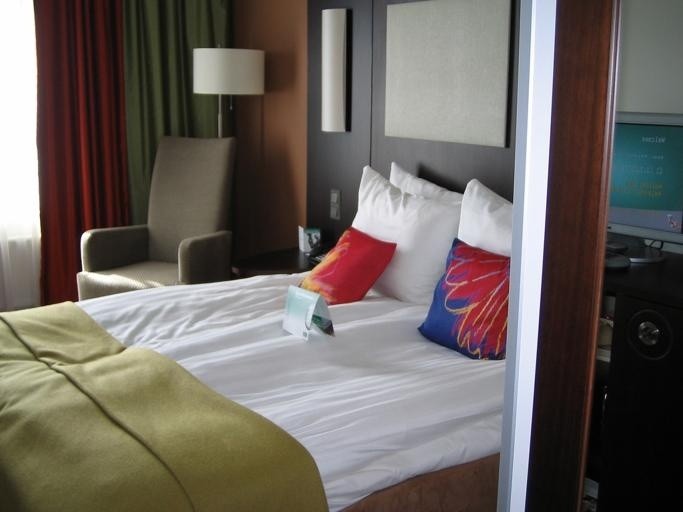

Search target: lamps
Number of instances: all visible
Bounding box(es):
[191,44,265,138]
[320,8,346,133]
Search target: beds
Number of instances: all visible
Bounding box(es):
[0,273,506,512]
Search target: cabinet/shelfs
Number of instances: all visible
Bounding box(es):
[584,245,682,511]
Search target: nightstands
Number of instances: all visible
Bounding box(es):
[230,246,314,277]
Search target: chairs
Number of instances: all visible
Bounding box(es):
[75,135,239,302]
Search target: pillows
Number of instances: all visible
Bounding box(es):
[417,237,510,359]
[298,226,397,305]
[387,161,463,204]
[458,178,513,257]
[352,164,461,305]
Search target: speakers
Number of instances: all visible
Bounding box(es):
[597,294,683,512]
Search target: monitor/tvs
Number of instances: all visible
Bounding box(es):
[607,111,683,264]
